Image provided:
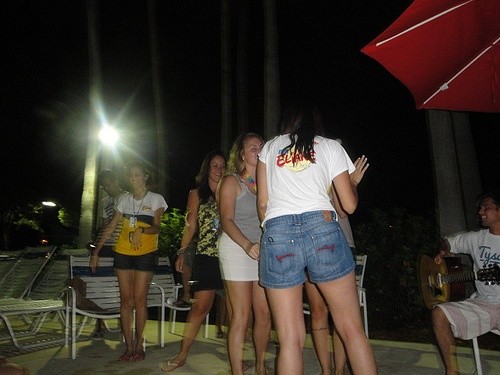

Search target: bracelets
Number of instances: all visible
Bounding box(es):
[139,226,144,233]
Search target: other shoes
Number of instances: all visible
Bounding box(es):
[118,350,145,362]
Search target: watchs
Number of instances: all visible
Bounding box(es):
[177,252,184,255]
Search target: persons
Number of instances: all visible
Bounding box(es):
[89,155,169,362]
[159,103,378,375]
[431,194,500,375]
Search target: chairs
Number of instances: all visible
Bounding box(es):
[303,255,368,338]
[0,245,210,360]
[469,291,500,375]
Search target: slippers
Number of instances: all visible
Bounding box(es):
[161,360,186,372]
[173,299,192,306]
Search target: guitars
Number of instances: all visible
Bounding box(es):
[419,253,500,309]
